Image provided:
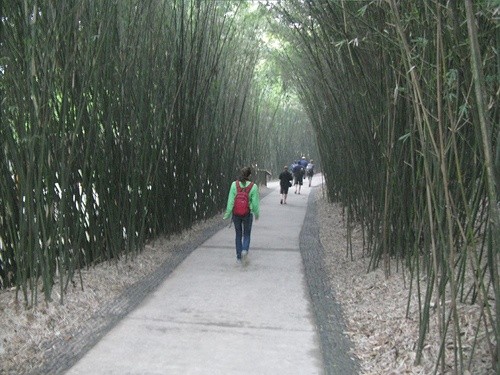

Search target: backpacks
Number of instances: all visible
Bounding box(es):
[232,179,254,217]
[306,169,313,176]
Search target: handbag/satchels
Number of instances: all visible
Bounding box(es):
[300,168,304,177]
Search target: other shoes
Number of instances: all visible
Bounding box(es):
[235,249,250,266]
[298,193,300,194]
[295,190,297,193]
[284,202,286,205]
[280,198,283,205]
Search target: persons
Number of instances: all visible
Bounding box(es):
[292,161,305,195]
[279,166,293,204]
[306,159,314,187]
[252,161,258,183]
[300,157,308,179]
[289,160,298,186]
[223,167,259,266]
